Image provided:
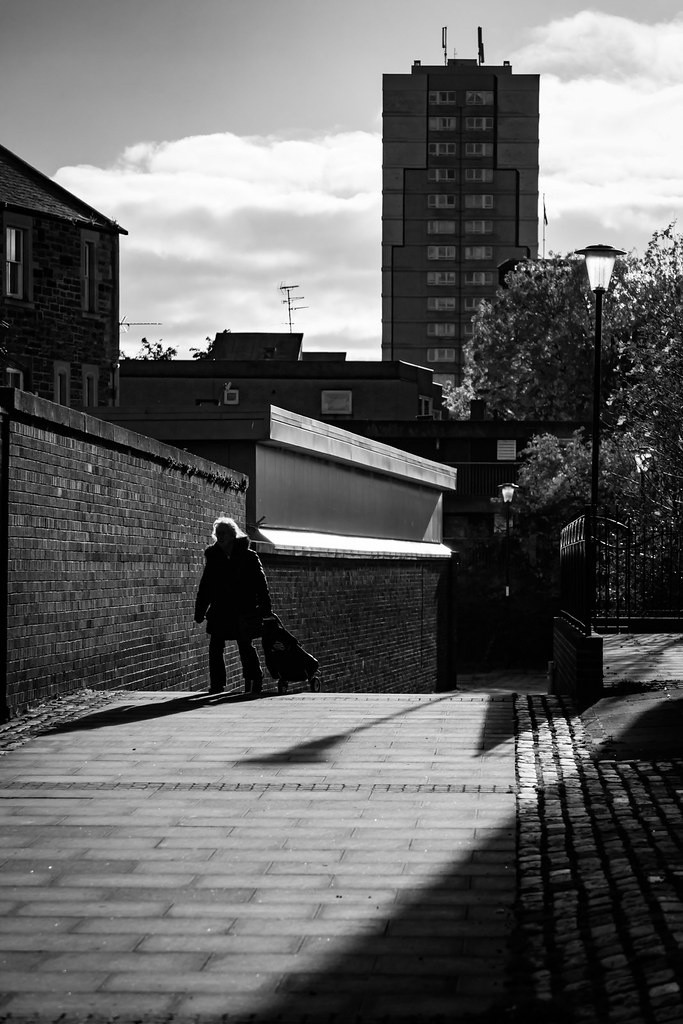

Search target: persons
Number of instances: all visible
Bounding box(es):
[194,516,274,692]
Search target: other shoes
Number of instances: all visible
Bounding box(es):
[209,682,225,694]
[245,678,262,693]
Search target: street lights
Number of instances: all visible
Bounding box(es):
[573,244,627,636]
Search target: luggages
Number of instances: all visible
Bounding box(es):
[262,611,321,695]
